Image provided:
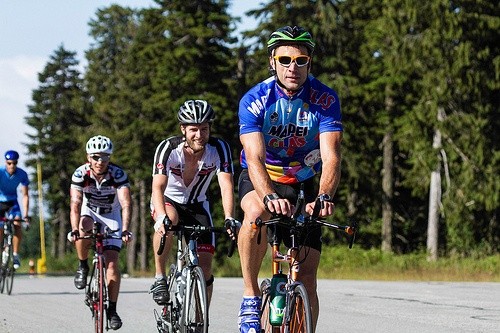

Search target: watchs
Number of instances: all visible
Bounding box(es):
[317,194,332,202]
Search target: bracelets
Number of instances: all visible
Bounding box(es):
[264,192,281,205]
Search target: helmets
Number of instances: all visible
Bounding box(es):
[85,134,114,154]
[177,99,216,125]
[5,150,20,160]
[267,24,317,52]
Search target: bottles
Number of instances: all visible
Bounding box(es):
[268,271,288,327]
[179,268,187,297]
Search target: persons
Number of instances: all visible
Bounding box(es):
[70,135,132,329]
[149,100,242,333]
[236,25,342,333]
[0,151,30,271]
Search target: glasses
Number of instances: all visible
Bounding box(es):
[89,155,111,162]
[7,162,18,165]
[272,55,310,67]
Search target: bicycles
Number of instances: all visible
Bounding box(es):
[70,221,132,333]
[0,216,32,296]
[153,214,239,333]
[250,184,358,333]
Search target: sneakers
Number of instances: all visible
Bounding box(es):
[238,295,264,333]
[149,278,170,302]
[106,308,123,330]
[74,265,90,289]
[13,255,21,269]
